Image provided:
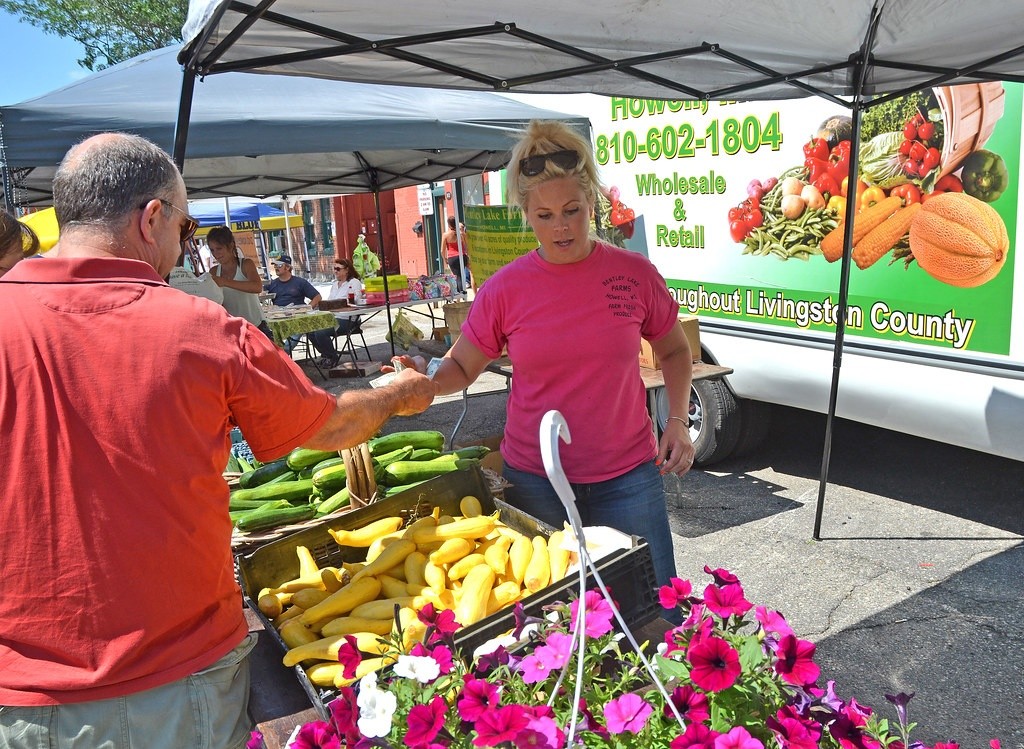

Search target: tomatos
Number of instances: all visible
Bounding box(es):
[728,197,763,243]
[610,199,635,240]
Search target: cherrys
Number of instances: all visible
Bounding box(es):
[899,106,940,177]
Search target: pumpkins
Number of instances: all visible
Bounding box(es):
[908,192,1009,288]
[816,115,852,154]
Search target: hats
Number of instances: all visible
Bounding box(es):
[271,255,291,265]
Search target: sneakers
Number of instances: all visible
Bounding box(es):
[313,358,326,367]
[321,358,338,369]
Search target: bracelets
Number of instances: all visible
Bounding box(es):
[665,415,691,429]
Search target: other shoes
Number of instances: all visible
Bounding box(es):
[466,282,470,288]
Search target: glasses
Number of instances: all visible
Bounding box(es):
[274,264,286,268]
[140,198,200,242]
[518,149,579,176]
[333,267,346,271]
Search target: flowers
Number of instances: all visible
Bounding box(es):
[242,564,1006,749]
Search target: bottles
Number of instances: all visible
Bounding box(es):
[348,286,354,305]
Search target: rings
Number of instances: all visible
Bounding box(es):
[686,458,694,464]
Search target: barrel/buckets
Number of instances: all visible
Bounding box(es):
[442,301,472,345]
[432,327,448,341]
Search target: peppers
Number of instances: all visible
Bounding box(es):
[889,173,963,209]
[803,135,886,220]
[961,149,1008,203]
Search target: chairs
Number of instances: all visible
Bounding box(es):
[319,314,372,369]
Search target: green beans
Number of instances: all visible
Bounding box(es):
[595,196,628,249]
[741,174,838,261]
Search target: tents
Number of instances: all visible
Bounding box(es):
[0,40,609,296]
[152,1,1021,545]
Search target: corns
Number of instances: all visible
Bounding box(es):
[852,203,923,270]
[820,195,901,263]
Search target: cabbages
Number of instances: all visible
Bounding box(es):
[858,131,941,195]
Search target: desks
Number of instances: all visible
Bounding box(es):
[409,340,733,508]
[330,293,467,379]
[266,310,338,381]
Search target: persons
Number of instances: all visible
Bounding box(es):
[0,207,40,278]
[0,134,445,748]
[262,256,322,360]
[379,118,697,632]
[307,258,364,368]
[205,226,277,346]
[470,271,478,298]
[438,214,469,295]
[459,222,471,290]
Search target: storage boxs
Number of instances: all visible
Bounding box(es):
[366,289,409,305]
[233,462,662,729]
[365,274,408,293]
[638,316,701,371]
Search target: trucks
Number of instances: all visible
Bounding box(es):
[592,77,1024,473]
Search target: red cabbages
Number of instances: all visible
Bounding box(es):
[860,90,933,143]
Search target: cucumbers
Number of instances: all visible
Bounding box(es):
[258,496,570,686]
[224,430,492,532]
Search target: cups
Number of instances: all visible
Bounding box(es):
[444,334,451,347]
